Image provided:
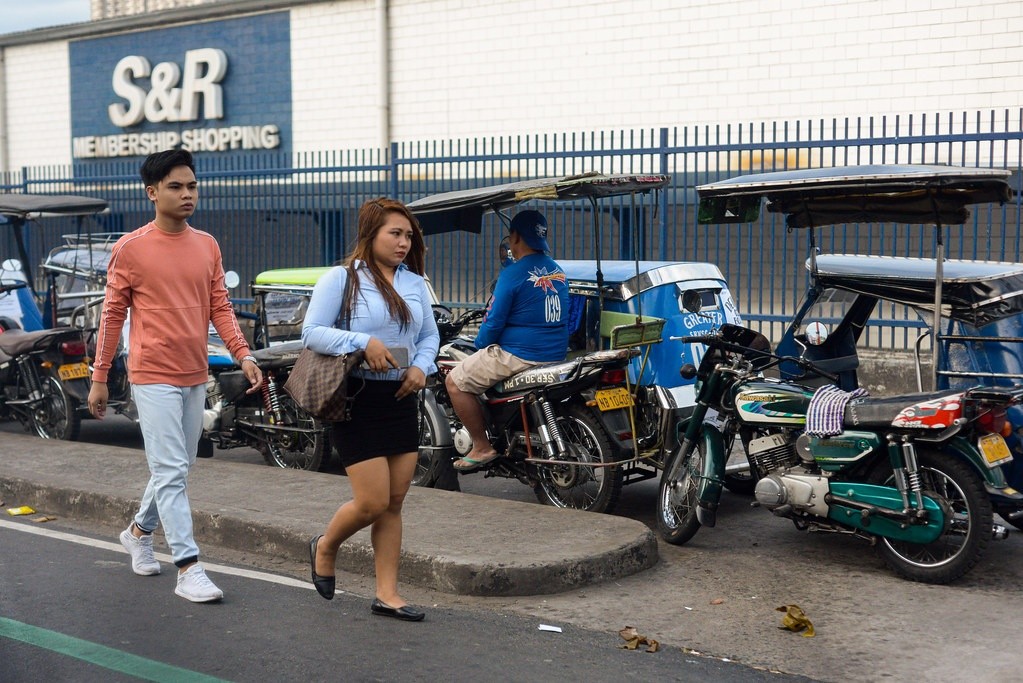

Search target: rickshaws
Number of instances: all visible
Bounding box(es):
[407,169,746,515]
[197,266,340,472]
[659,161,1022,581]
[0,192,132,440]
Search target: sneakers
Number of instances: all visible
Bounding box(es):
[174,561,224,603]
[119,521,161,576]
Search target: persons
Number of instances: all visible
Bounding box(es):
[87,149,263,603]
[282,197,441,621]
[443,210,571,471]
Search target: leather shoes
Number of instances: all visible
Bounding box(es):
[309,535,335,600]
[371,597,425,621]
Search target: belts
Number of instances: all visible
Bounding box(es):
[349,368,409,381]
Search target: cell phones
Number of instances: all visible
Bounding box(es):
[364,347,409,371]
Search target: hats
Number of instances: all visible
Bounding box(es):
[511,210,551,253]
[502,242,509,250]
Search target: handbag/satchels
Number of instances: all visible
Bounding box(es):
[281,267,365,424]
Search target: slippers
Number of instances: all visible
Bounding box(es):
[453,453,500,470]
